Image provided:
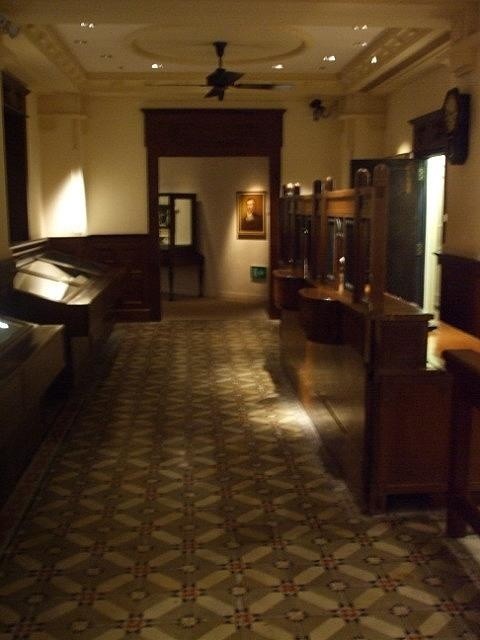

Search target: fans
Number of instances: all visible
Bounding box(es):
[145,41,275,101]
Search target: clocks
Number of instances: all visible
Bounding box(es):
[442,88,471,166]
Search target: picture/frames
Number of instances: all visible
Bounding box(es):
[236,191,266,238]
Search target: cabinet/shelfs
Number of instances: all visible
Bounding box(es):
[439,347,480,538]
[298,163,480,514]
[158,193,204,302]
[272,181,349,390]
